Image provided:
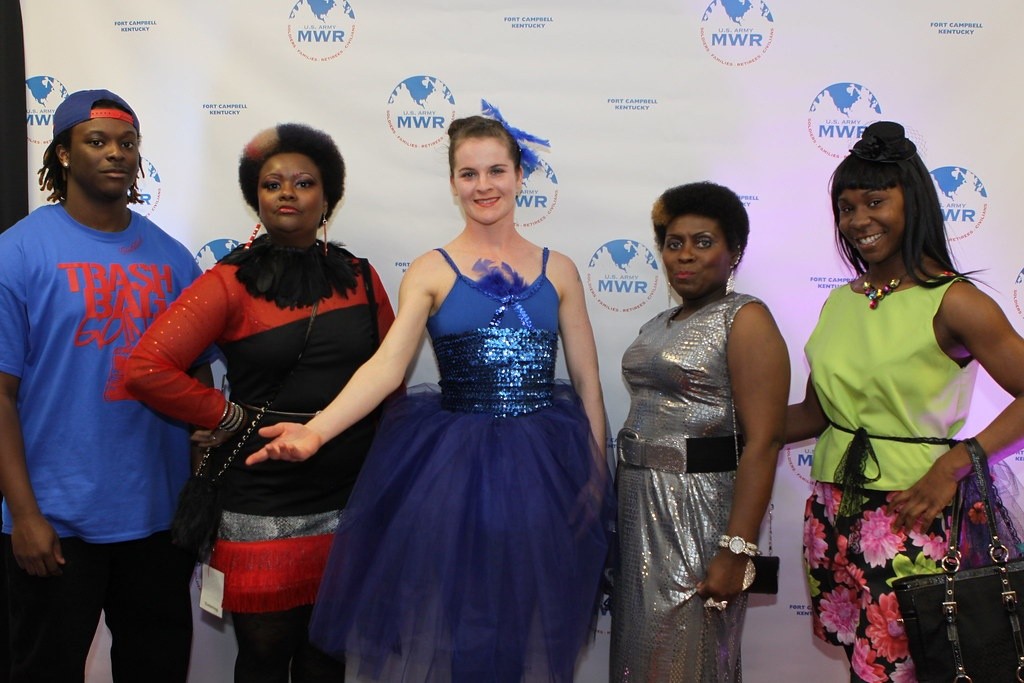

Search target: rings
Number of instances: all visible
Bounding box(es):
[213,436,215,440]
[703,597,727,611]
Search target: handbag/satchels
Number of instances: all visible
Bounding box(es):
[740,554,780,595]
[891,437,1024,683]
[164,476,222,564]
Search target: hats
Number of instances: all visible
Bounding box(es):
[52,89,140,138]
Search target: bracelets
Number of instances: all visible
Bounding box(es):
[217,400,243,433]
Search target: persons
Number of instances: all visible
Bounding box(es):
[0,88,212,683]
[612,182,791,683]
[246,116,609,683]
[775,120,1024,683]
[121,123,403,683]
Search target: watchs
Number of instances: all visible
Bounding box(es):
[715,535,757,558]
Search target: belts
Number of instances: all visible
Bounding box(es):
[616,427,743,475]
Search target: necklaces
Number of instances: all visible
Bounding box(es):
[859,258,924,309]
